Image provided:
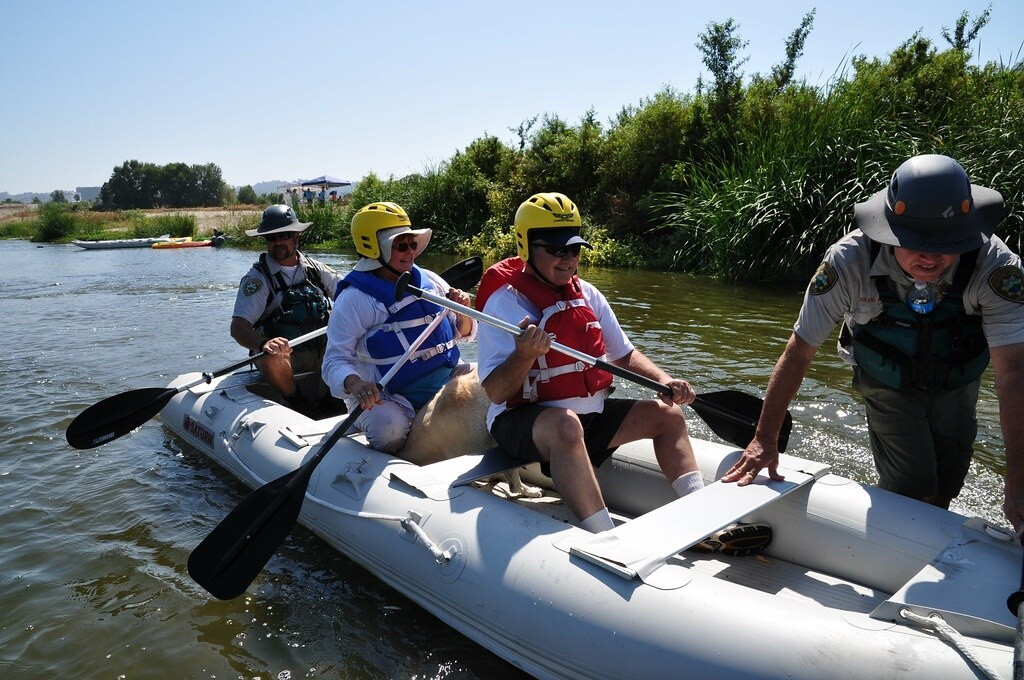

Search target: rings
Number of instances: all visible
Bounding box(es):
[367,391,373,395]
[362,393,366,396]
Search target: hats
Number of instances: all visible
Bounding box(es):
[529,228,594,252]
[854,154,1004,256]
[245,204,314,237]
[352,226,432,272]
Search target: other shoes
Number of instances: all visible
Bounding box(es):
[281,384,311,413]
[687,522,773,556]
[310,391,340,416]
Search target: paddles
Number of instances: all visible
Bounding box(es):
[396,268,794,456]
[187,294,457,600]
[65,254,482,451]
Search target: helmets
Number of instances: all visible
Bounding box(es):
[350,201,411,260]
[513,192,582,262]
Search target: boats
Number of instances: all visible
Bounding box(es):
[164,358,1024,680]
[70,234,170,249]
[152,240,211,249]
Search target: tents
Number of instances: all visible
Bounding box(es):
[277,175,304,203]
[301,175,352,204]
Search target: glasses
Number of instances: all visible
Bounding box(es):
[264,231,301,243]
[529,242,581,258]
[392,241,417,252]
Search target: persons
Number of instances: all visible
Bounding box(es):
[322,202,479,455]
[722,154,1024,547]
[302,187,336,204]
[474,192,773,559]
[231,203,344,420]
